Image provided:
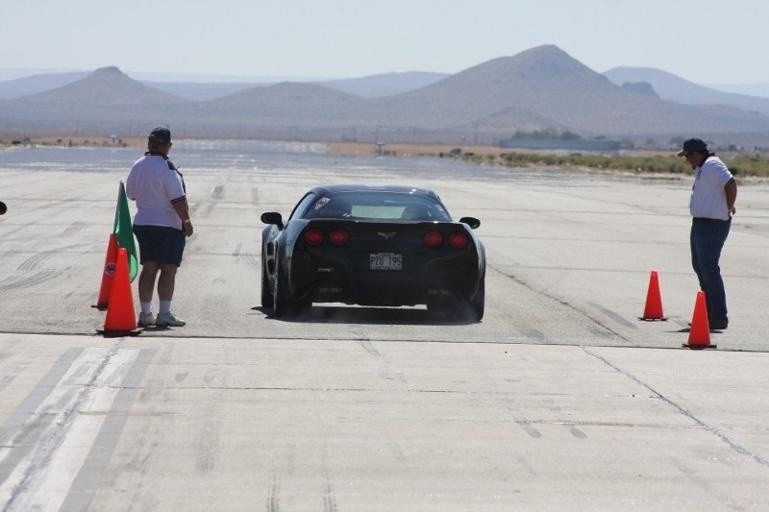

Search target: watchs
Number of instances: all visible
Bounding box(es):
[182,219,190,223]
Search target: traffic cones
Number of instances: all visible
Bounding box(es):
[90,232,123,310]
[679,290,717,349]
[94,244,144,339]
[637,270,669,322]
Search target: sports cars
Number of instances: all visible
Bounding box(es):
[254,180,488,325]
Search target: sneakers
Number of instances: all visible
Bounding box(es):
[687,318,729,330]
[138,312,156,327]
[155,312,186,327]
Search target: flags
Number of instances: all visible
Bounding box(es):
[113,182,139,283]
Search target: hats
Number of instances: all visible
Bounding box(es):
[149,126,172,144]
[678,139,708,156]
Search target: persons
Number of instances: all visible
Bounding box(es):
[124,127,194,328]
[676,137,738,330]
